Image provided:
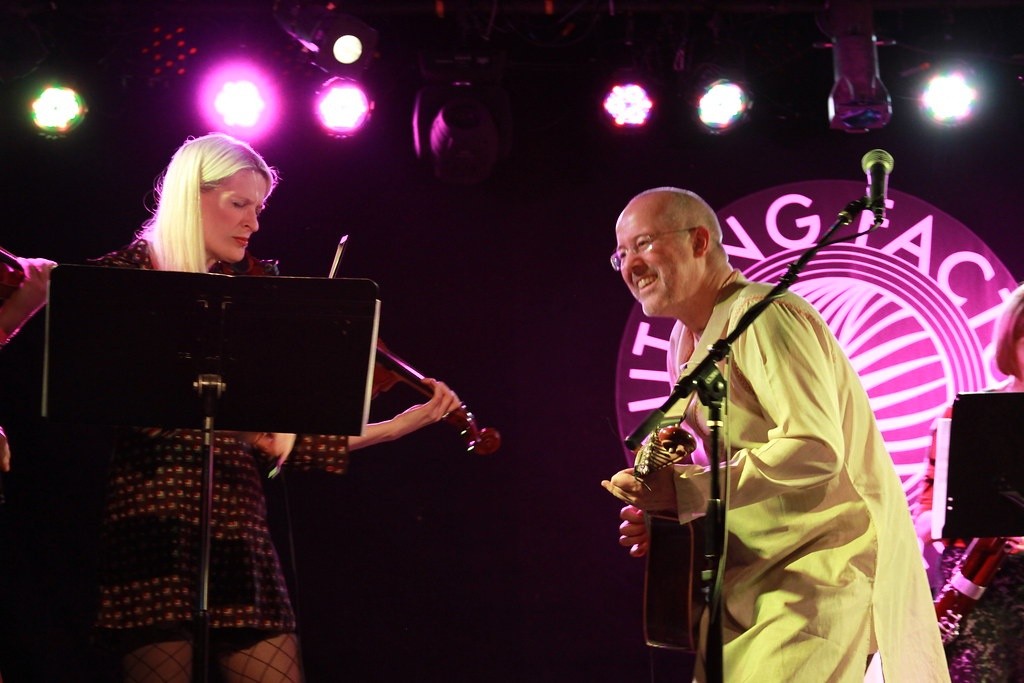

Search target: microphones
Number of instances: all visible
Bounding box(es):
[860,148,895,220]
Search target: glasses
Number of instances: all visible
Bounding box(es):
[609,228,696,272]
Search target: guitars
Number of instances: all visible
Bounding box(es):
[635,425,722,654]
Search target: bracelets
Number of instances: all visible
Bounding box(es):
[236,432,267,445]
[0,329,10,345]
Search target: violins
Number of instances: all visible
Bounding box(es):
[207,250,502,456]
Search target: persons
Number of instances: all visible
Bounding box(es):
[86,134,461,683]
[934,284,1024,683]
[601,186,952,683]
[0,257,59,472]
